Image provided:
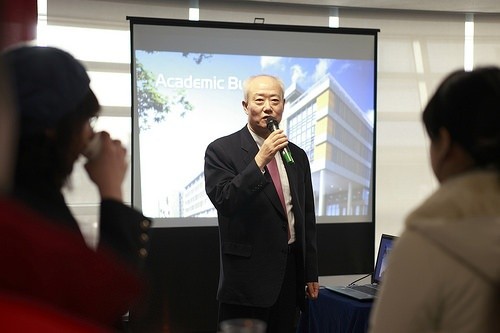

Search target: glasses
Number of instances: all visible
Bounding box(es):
[89,116,98,129]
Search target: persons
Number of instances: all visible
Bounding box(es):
[204,75,319,333]
[367,65,499,333]
[0,44,153,332]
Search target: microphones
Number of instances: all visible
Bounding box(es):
[266,116,295,166]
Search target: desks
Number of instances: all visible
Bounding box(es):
[303,284,381,333]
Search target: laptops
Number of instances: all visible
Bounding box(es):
[326,234,399,302]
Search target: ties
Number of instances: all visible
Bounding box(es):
[265,155,291,242]
